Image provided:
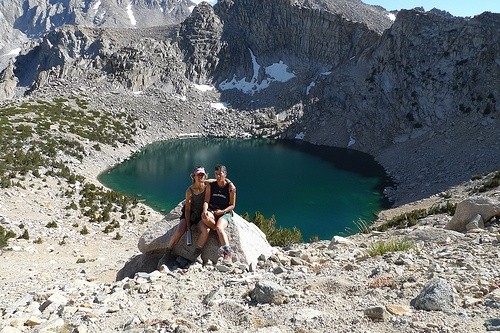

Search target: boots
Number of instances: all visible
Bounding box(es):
[192,247,203,264]
[157,247,173,268]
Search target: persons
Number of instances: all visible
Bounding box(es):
[161,166,236,263]
[201,164,236,263]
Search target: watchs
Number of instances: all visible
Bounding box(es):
[222,209,227,215]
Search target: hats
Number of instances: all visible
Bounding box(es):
[194,168,207,177]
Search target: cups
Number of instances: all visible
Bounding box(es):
[187,229,192,246]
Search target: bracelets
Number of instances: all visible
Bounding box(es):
[229,181,232,184]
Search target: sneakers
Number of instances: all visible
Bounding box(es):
[214,230,221,247]
[223,246,232,266]
